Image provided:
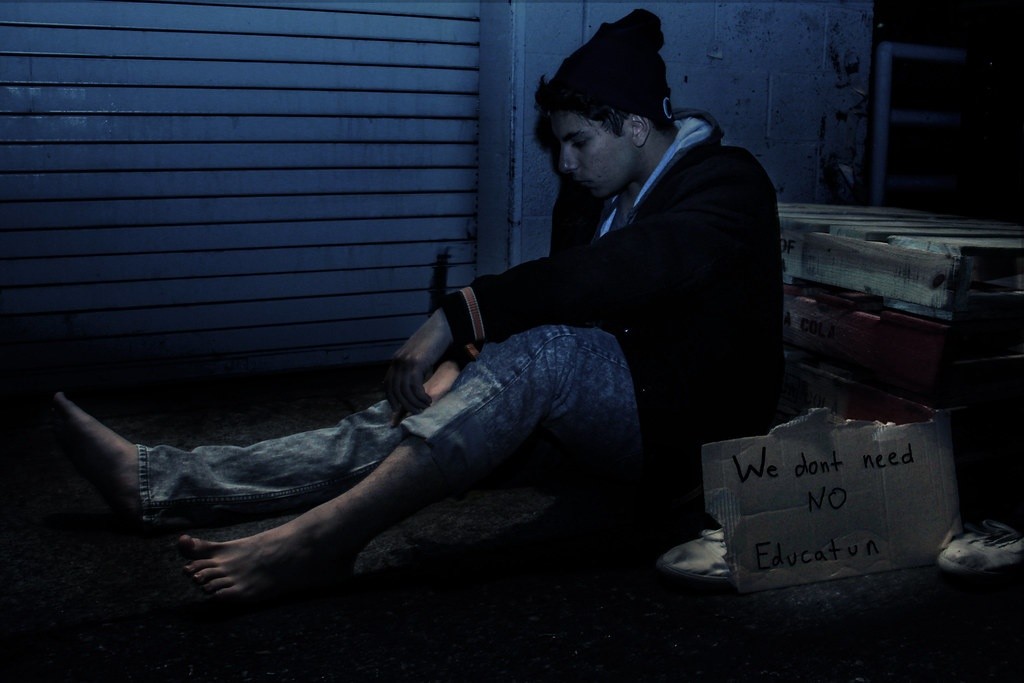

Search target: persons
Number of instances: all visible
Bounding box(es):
[51,6,786,615]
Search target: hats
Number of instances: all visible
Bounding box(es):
[545,9,676,123]
